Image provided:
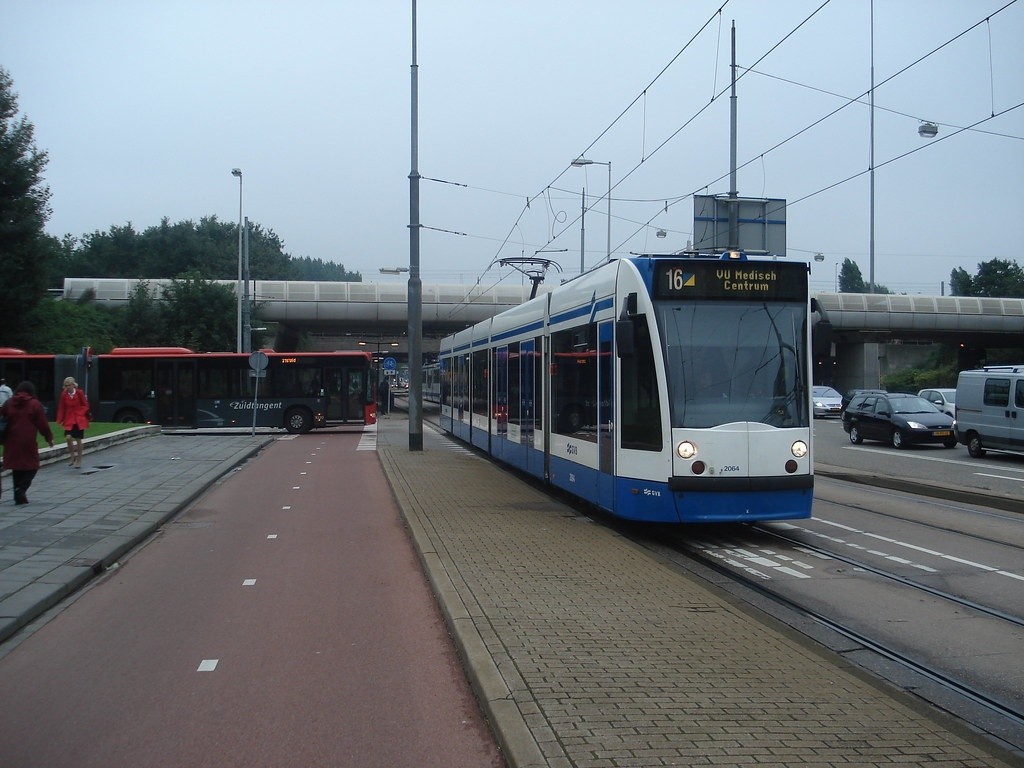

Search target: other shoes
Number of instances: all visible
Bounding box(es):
[14,487,28,505]
[68,457,76,467]
[74,460,81,468]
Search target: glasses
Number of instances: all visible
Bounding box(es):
[64,384,72,387]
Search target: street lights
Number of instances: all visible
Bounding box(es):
[378,265,423,450]
[231,167,244,354]
[571,158,612,260]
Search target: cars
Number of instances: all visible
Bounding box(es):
[812,386,844,421]
[918,388,957,419]
[844,388,888,412]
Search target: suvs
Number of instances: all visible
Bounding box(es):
[840,392,957,451]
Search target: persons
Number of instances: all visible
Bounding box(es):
[0,379,12,410]
[0,381,54,505]
[55,376,89,469]
[380,376,390,415]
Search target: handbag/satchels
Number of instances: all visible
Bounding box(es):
[78,390,93,421]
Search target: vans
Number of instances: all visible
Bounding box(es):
[954,366,1024,460]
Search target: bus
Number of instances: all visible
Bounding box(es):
[0,345,378,435]
[397,366,409,389]
[421,362,442,406]
[438,255,837,524]
